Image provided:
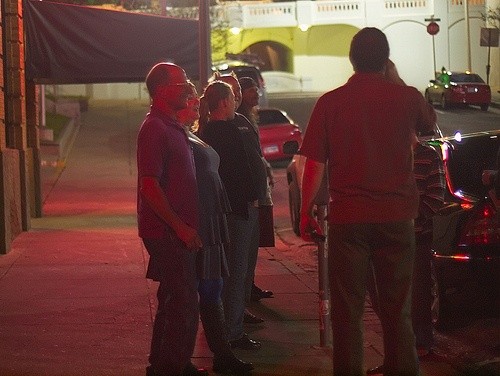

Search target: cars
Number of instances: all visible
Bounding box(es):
[424,69,491,112]
[211,59,264,97]
[413,122,443,139]
[412,129,500,335]
[254,107,303,162]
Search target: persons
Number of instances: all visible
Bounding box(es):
[177,65,275,376]
[412,130,445,361]
[298,28,436,376]
[135,63,208,376]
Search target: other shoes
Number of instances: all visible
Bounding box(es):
[367,364,384,375]
[231,334,262,351]
[212,356,254,373]
[255,287,273,298]
[185,360,208,376]
[244,312,264,323]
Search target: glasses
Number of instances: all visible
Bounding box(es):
[167,79,190,88]
